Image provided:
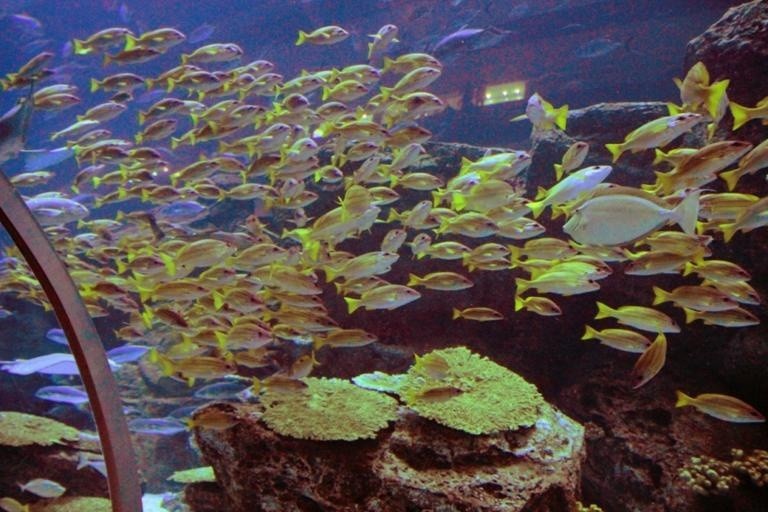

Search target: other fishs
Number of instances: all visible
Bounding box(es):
[0,25,768,405]
